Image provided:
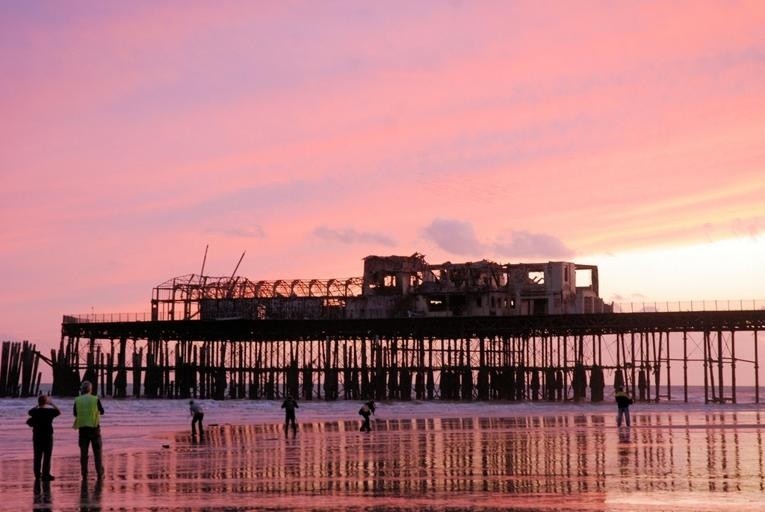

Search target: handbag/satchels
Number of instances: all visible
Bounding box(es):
[26,418,33,427]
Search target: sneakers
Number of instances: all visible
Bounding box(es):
[34,473,55,481]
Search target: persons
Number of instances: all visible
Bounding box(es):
[357,400,375,432]
[26,395,62,481]
[281,394,300,430]
[187,400,204,435]
[72,381,106,480]
[614,385,634,427]
